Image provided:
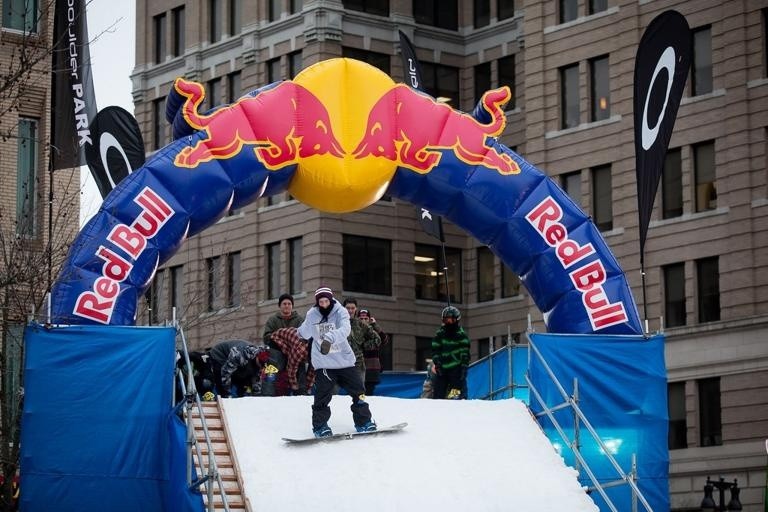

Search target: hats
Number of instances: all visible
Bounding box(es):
[260,351,271,365]
[314,285,333,302]
[278,294,294,307]
[357,309,371,318]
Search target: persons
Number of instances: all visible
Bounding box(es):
[173,327,317,402]
[357,309,388,395]
[432,306,471,399]
[421,359,436,399]
[325,297,382,401]
[297,285,376,436]
[263,293,303,337]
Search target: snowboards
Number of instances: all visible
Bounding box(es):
[281,422,408,442]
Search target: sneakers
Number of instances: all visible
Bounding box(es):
[314,423,332,436]
[354,421,377,430]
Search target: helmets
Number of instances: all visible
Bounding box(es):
[441,306,461,333]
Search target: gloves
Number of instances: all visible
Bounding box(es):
[460,365,467,381]
[435,361,443,377]
